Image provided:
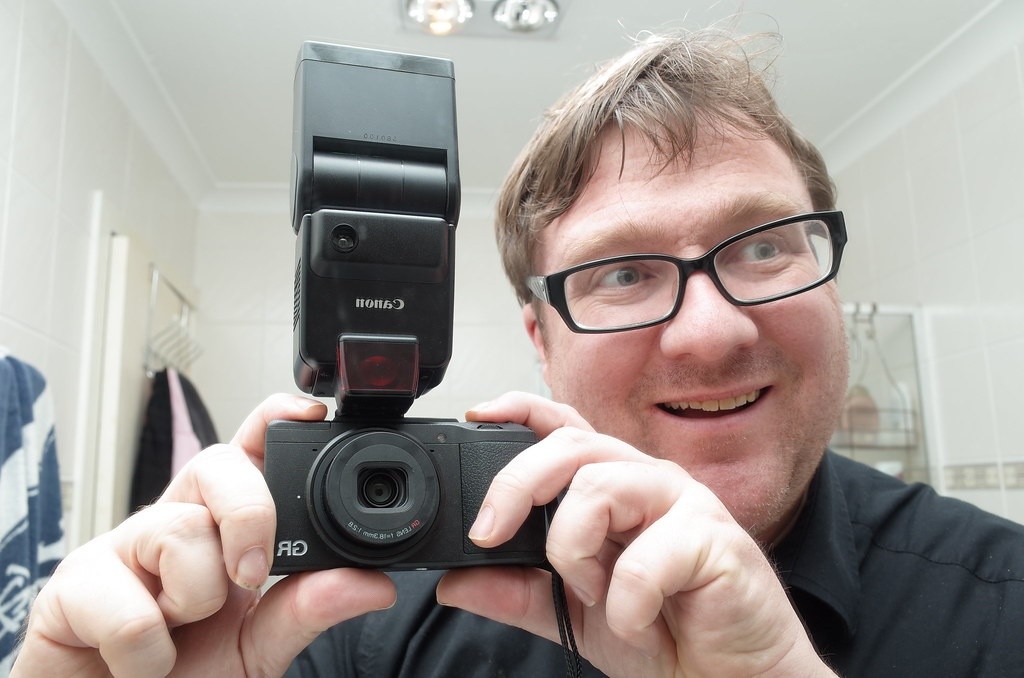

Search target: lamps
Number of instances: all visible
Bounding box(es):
[400,0,571,43]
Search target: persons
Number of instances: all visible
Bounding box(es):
[0,1,1021,677]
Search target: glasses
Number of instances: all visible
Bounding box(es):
[525,211,849,334]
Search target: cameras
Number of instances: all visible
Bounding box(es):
[261,40,567,573]
